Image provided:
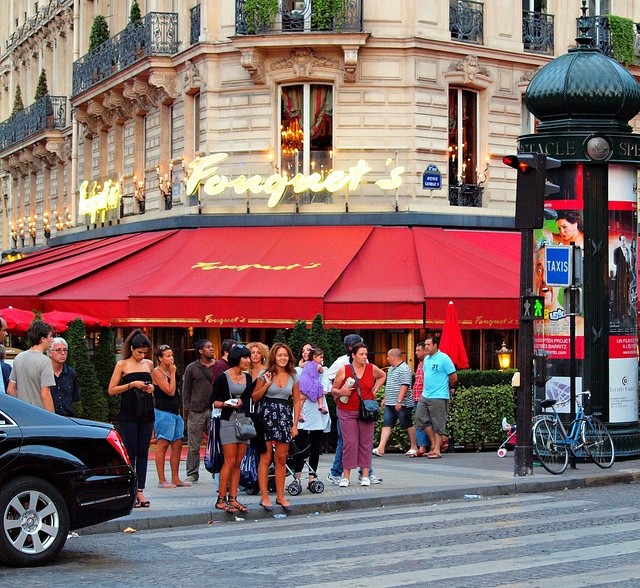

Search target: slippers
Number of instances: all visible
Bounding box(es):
[372,447,384,457]
[428,452,441,457]
[411,450,424,458]
[442,437,450,453]
[422,450,430,455]
[404,448,415,456]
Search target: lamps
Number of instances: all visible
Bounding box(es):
[497,342,513,369]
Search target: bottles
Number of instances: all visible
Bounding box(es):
[464,494,482,501]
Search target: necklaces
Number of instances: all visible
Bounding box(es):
[232,371,243,385]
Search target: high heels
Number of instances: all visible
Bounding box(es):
[137,490,151,508]
[133,500,140,508]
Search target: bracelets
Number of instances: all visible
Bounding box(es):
[372,388,377,391]
[127,383,130,390]
[221,402,224,408]
[397,400,402,403]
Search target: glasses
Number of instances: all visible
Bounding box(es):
[234,343,245,350]
[160,343,171,350]
[51,347,68,354]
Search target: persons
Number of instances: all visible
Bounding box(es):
[534,244,585,337]
[612,234,630,319]
[252,343,301,512]
[371,349,418,457]
[630,238,637,273]
[415,336,458,459]
[0,317,9,394]
[210,344,253,514]
[333,343,387,488]
[285,342,332,490]
[298,348,328,423]
[327,334,383,487]
[48,337,81,418]
[543,210,584,248]
[0,344,12,391]
[182,339,220,484]
[150,344,192,489]
[408,342,449,458]
[246,341,269,386]
[107,328,155,508]
[7,322,57,414]
[212,339,237,382]
[501,417,517,432]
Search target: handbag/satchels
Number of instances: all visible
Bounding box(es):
[359,398,379,420]
[234,415,258,439]
[240,446,258,487]
[205,419,224,473]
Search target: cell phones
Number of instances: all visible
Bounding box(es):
[145,381,151,385]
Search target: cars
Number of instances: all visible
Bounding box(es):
[0,392,139,567]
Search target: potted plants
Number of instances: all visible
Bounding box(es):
[120,0,145,67]
[89,14,116,81]
[33,69,54,128]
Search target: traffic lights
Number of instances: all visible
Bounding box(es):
[520,295,544,320]
[544,152,561,221]
[502,152,539,174]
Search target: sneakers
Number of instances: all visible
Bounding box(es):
[327,472,340,484]
[158,481,177,488]
[185,474,198,481]
[171,480,191,486]
[362,476,370,485]
[357,474,383,484]
[276,497,293,510]
[318,405,328,414]
[339,478,349,486]
[298,416,305,422]
[260,499,273,512]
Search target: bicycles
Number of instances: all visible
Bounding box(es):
[531,391,615,475]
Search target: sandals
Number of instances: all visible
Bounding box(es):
[228,493,247,512]
[214,495,238,513]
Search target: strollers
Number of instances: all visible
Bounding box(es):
[497,430,516,458]
[244,432,324,496]
